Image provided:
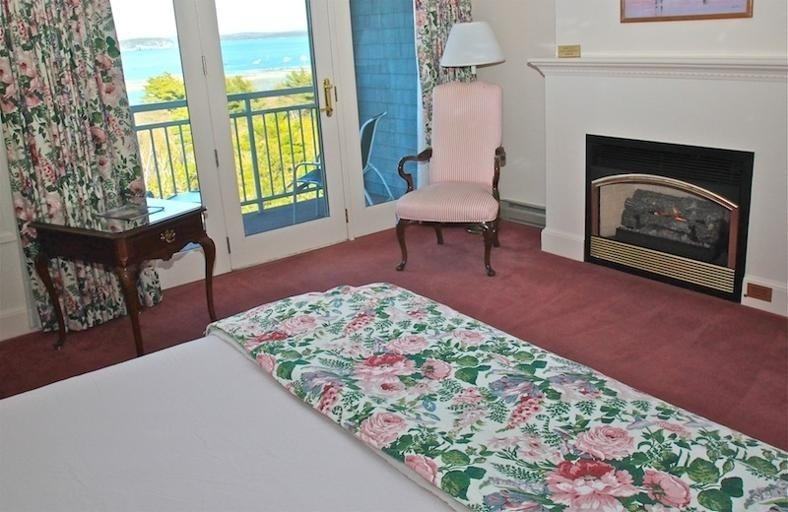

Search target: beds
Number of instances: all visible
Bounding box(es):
[1,279,787,510]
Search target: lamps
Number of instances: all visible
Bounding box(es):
[439,21,506,236]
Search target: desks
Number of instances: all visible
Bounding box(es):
[27,193,217,357]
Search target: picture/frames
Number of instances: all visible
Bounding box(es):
[620,0,754,24]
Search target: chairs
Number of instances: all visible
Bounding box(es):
[395,83,507,277]
[292,111,394,226]
